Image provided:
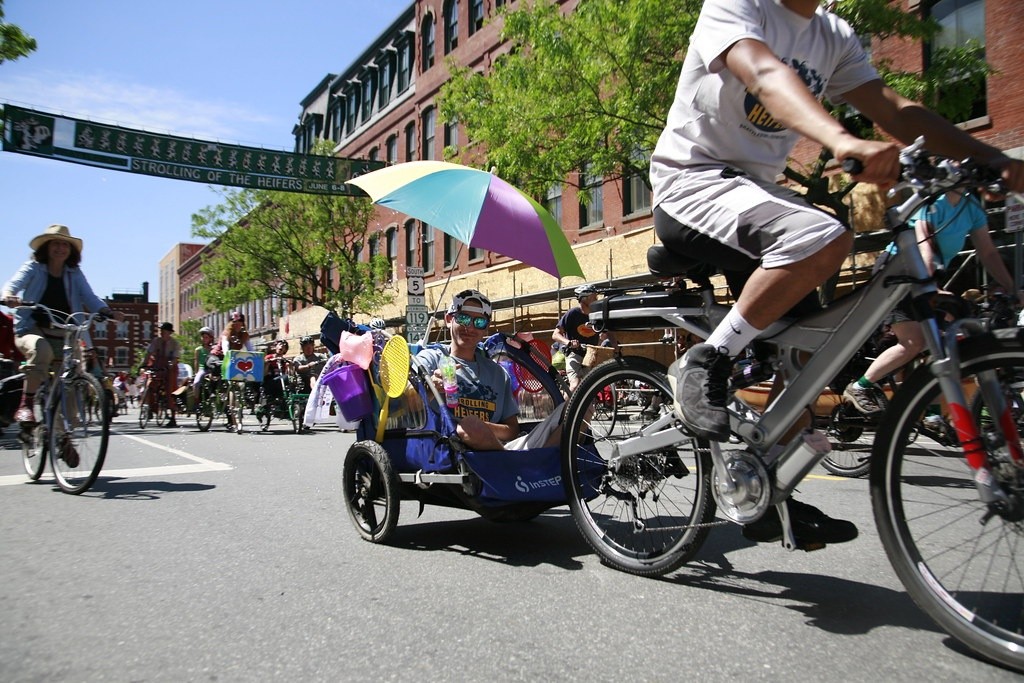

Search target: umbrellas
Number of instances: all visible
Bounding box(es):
[342,160,585,346]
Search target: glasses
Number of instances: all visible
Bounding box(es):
[452,314,489,330]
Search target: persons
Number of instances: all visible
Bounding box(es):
[552,284,610,401]
[649,0,1024,544]
[842,159,1024,448]
[388,290,595,451]
[97,312,386,433]
[0,225,124,469]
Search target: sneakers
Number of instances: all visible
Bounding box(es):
[741,495,858,544]
[843,379,880,413]
[668,341,741,443]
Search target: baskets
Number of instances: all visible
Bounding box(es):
[582,345,615,367]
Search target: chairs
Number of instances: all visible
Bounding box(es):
[319,309,607,525]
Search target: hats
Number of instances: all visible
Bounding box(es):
[229,313,244,321]
[29,224,82,253]
[445,290,491,315]
[158,323,174,332]
[199,327,213,336]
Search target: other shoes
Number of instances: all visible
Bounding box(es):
[59,438,79,468]
[13,408,34,425]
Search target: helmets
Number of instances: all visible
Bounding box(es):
[574,284,595,303]
[300,335,313,345]
[274,339,288,354]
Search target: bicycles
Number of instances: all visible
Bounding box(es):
[0,295,331,495]
[559,134,1024,676]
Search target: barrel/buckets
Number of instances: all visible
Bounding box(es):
[322,362,374,423]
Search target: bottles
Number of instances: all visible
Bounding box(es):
[439,358,458,409]
